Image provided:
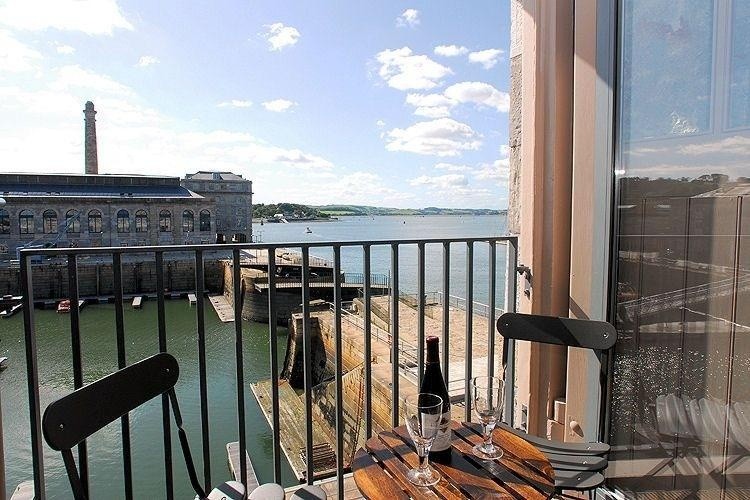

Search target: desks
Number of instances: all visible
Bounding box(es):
[351,420,557,500]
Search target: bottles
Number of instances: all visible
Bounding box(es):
[417,335,453,462]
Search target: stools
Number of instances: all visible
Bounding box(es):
[629,375,750,491]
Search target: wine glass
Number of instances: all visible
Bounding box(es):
[470,375,506,462]
[403,393,445,487]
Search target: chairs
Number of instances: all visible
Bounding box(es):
[42,352,327,500]
[495,311,618,499]
